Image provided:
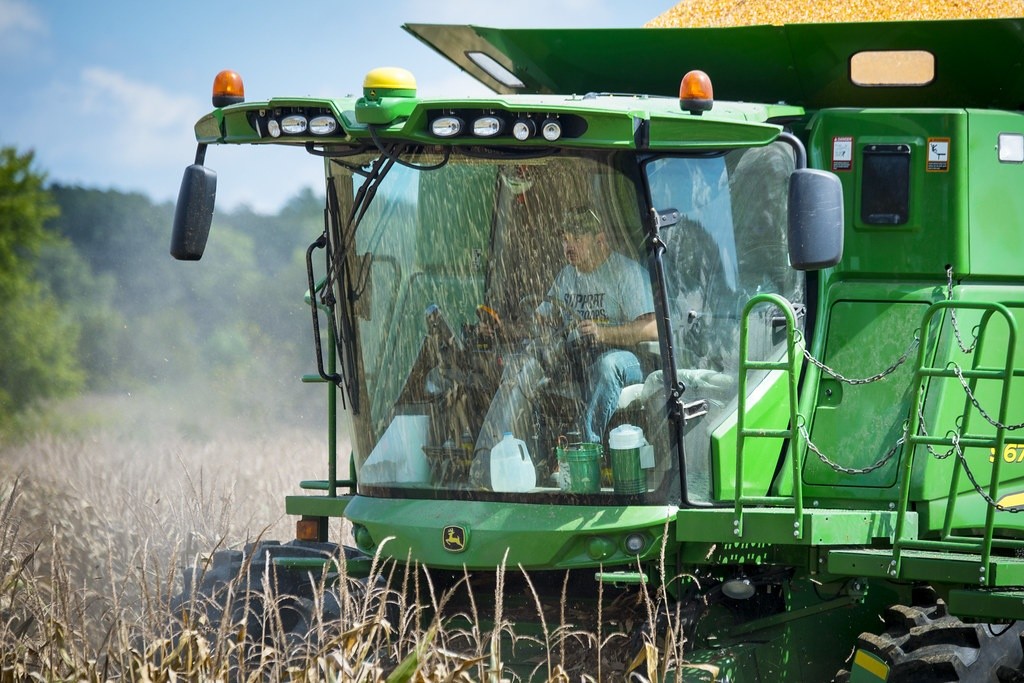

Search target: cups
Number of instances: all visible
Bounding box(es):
[566,431,583,451]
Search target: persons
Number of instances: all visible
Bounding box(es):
[478,205,658,445]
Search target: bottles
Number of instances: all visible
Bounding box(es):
[490,433,536,491]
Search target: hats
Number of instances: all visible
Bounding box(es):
[551,206,605,235]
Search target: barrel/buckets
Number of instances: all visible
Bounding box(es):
[610,424,648,494]
[557,443,603,495]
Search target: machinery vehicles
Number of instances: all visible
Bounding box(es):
[171,18,1024,683]
